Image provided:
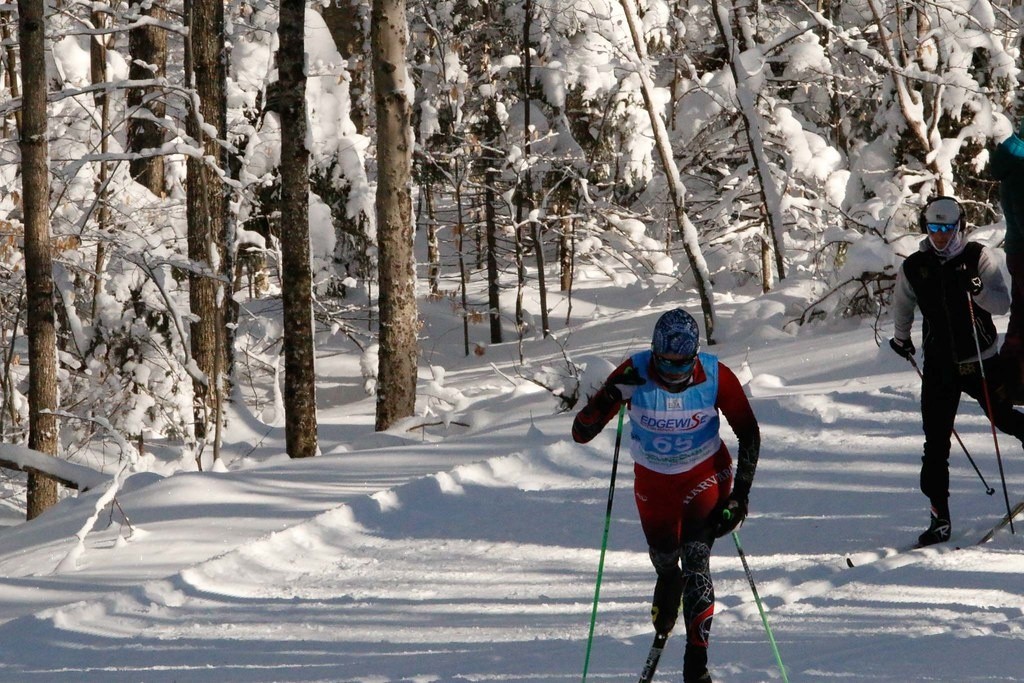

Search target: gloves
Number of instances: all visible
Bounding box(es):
[889,335,915,362]
[593,368,647,415]
[713,478,751,539]
[949,262,984,295]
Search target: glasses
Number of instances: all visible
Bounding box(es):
[926,215,960,233]
[655,354,695,369]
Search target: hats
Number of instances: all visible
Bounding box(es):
[924,199,960,229]
[652,308,699,355]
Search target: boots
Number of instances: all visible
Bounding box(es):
[651,565,683,634]
[918,511,951,546]
[683,645,713,683]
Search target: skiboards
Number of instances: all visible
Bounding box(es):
[845,501,1024,569]
[637,568,713,683]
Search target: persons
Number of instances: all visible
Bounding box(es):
[572,308,761,683]
[977,117,1024,404]
[891,197,1024,545]
